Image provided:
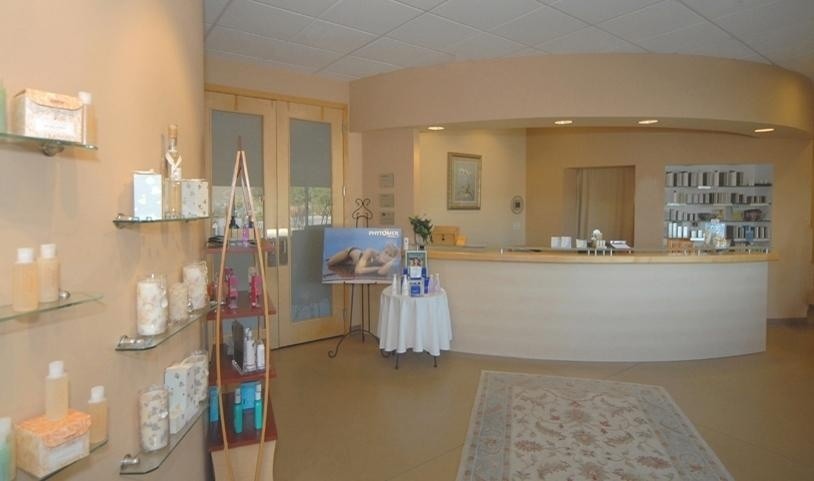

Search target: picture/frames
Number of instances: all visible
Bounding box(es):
[447,152,483,210]
[406,250,428,287]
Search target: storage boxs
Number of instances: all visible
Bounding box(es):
[10,88,87,143]
[14,407,90,478]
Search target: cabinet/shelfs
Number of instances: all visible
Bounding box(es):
[664,164,774,252]
[208,240,280,481]
[0,133,218,481]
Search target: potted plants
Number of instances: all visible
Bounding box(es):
[408,217,433,248]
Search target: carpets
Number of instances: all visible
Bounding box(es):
[457,369,735,481]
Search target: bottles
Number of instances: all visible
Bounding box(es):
[232,383,265,434]
[11,240,62,317]
[44,360,72,422]
[242,327,256,372]
[163,123,183,218]
[86,383,110,446]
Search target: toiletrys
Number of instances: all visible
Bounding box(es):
[233,394,242,433]
[254,391,262,430]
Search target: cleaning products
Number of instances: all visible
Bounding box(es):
[36,244,60,302]
[1,417,17,481]
[209,210,264,435]
[45,359,69,419]
[87,386,108,444]
[13,247,37,312]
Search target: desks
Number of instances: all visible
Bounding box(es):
[377,285,453,370]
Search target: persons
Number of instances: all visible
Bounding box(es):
[325,242,399,276]
